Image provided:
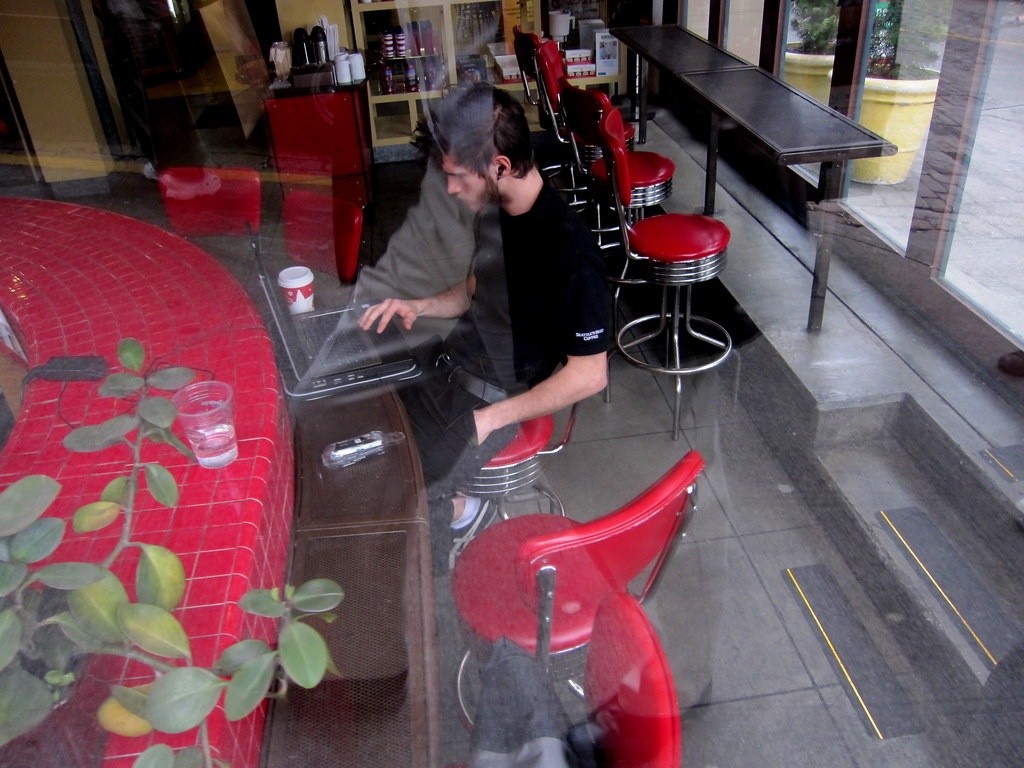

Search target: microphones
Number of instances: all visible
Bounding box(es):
[497,164,506,181]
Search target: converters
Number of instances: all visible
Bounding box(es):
[40,355,107,381]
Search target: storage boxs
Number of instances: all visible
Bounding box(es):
[594,29,619,77]
[579,19,605,48]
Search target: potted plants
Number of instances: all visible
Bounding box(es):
[827,0,940,185]
[782,0,839,106]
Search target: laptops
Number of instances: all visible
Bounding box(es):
[243,220,417,397]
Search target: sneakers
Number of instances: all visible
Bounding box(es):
[433,497,498,578]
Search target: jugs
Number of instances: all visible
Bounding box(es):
[293,28,315,64]
[309,26,329,63]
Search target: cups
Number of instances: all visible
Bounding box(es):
[277,266,315,315]
[333,52,366,84]
[170,381,239,470]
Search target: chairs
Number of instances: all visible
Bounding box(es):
[584,590,682,768]
[451,450,706,734]
[510,27,743,439]
[159,166,263,260]
[458,402,579,523]
[282,189,365,278]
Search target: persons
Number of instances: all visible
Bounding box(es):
[355,82,611,581]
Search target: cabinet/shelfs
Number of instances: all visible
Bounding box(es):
[349,1,628,149]
[265,78,371,179]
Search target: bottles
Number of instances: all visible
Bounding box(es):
[404,61,418,92]
[381,65,394,94]
[270,41,291,80]
[378,20,433,57]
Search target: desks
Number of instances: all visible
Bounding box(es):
[611,25,899,332]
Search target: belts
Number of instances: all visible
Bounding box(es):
[430,341,516,405]
